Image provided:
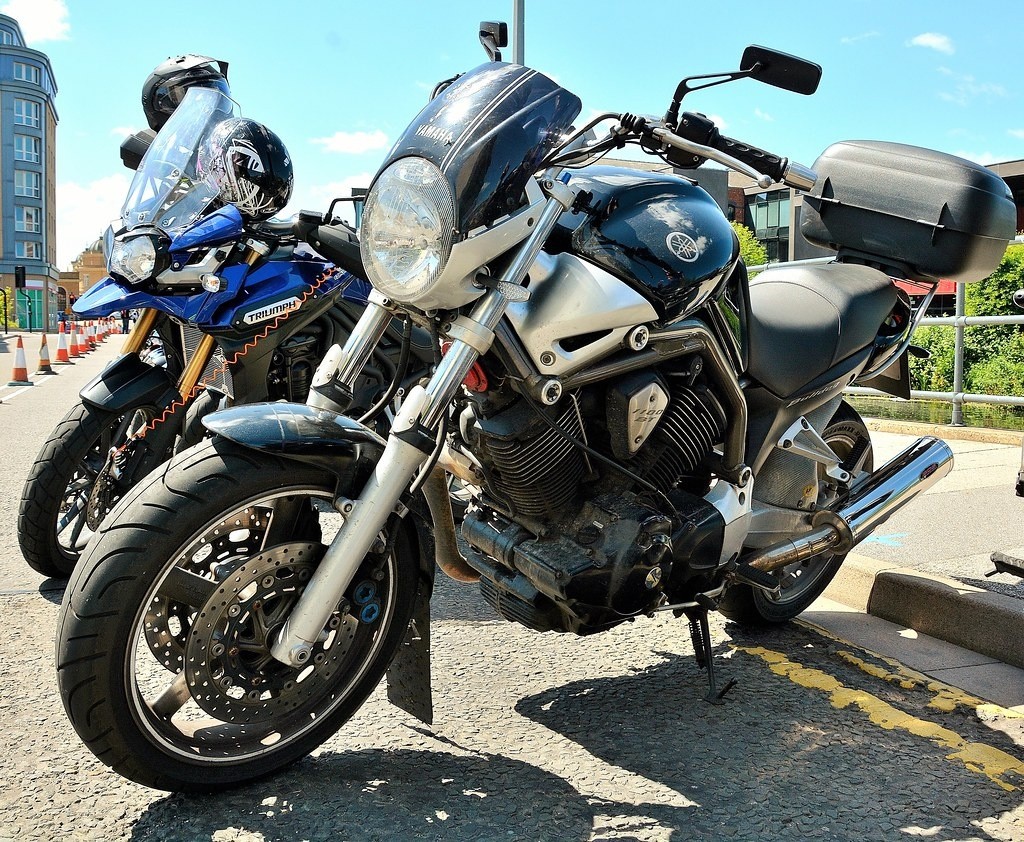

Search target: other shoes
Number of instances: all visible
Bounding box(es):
[119,332,129,334]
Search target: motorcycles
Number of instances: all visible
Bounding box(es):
[55,21,1022,798]
[9,51,467,588]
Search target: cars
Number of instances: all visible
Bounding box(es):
[107,309,139,319]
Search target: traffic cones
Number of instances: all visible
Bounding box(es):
[67,321,85,360]
[38,331,57,375]
[8,335,34,385]
[50,318,76,364]
[81,316,123,351]
[76,325,89,355]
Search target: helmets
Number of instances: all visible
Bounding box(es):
[142,53,232,134]
[196,116,294,224]
[120,129,172,178]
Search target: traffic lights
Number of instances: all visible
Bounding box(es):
[28,301,32,306]
[69,293,75,304]
[15,266,25,288]
